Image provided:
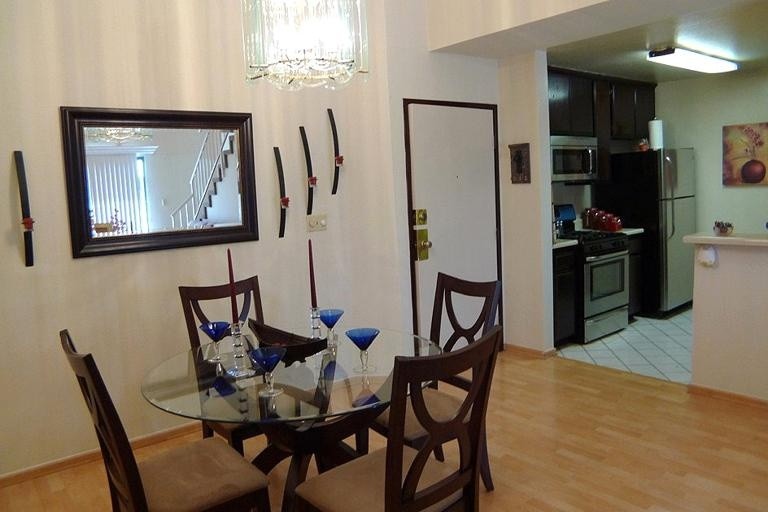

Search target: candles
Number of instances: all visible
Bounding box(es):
[226,247,240,325]
[308,238,320,309]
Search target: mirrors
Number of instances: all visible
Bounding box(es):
[58,104,260,259]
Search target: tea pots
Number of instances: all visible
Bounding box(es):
[582,208,592,228]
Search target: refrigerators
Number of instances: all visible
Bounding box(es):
[592,148,696,318]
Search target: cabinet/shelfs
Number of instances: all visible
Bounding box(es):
[549,72,595,135]
[627,232,647,317]
[611,84,654,140]
[553,245,579,347]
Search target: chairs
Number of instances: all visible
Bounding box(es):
[286,326,509,510]
[350,271,503,496]
[60,328,276,510]
[175,272,329,475]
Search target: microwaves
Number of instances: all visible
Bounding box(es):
[550,136,599,181]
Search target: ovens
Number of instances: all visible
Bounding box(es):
[581,250,631,344]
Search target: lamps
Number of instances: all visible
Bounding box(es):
[240,1,372,94]
[648,46,739,77]
[86,125,153,144]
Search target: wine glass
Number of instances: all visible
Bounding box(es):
[346,328,380,376]
[246,346,286,397]
[204,360,236,398]
[317,308,342,350]
[351,376,381,407]
[199,322,230,362]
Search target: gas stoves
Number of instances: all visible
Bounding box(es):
[562,230,629,255]
